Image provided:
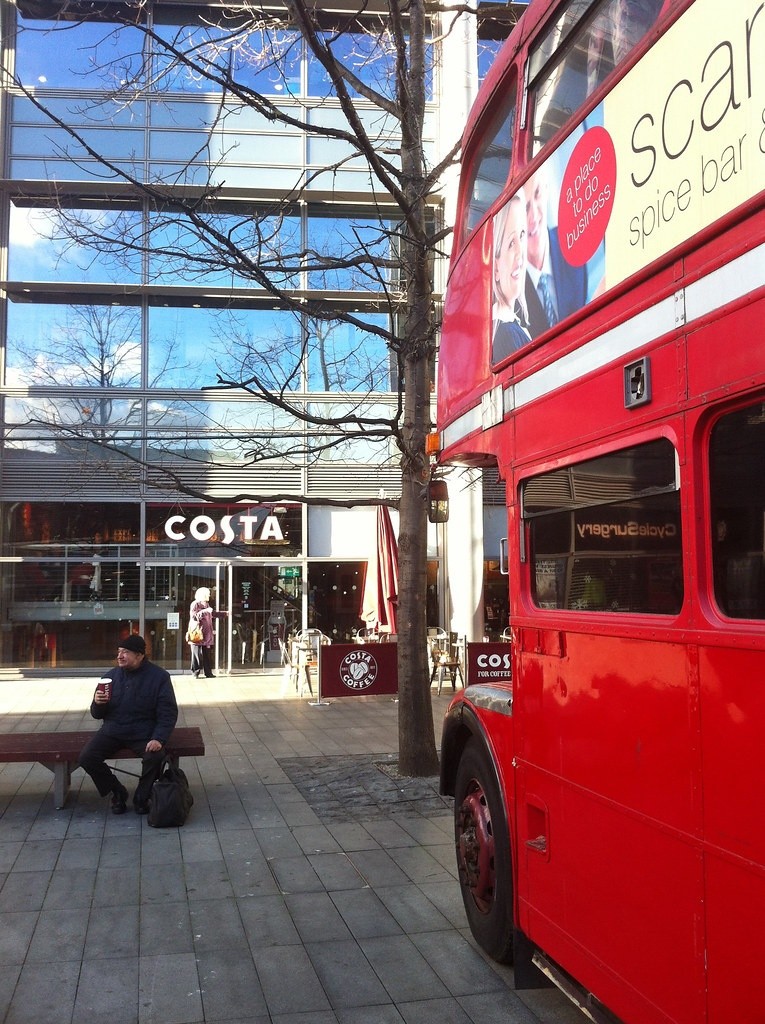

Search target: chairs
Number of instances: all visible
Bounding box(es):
[425,627,465,696]
[278,637,314,697]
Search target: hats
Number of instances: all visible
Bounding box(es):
[116,635,146,653]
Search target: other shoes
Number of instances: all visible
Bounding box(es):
[111,785,129,814]
[132,792,150,814]
[193,674,198,679]
[206,674,216,678]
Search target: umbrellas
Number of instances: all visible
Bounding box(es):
[361,489,400,639]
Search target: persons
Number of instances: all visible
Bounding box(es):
[80,634,178,814]
[492,172,607,365]
[190,587,229,678]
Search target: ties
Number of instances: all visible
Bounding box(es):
[537,274,557,330]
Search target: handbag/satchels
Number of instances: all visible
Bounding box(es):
[147,755,194,828]
[188,615,204,643]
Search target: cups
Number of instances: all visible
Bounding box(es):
[98,678,112,700]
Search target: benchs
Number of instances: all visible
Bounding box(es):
[0,727,205,811]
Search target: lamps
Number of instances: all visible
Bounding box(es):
[427,478,450,523]
[272,506,288,514]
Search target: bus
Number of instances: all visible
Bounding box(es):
[417,0,765,1024]
